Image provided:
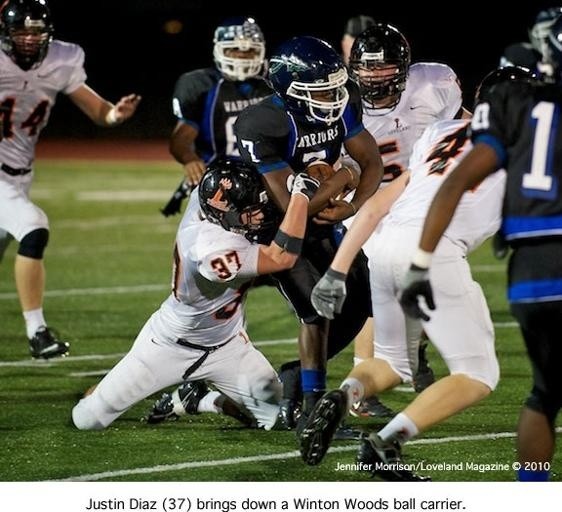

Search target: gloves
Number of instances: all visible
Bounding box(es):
[398,263,437,323]
[286,171,321,203]
[309,266,349,320]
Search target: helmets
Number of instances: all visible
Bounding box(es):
[197,156,271,241]
[347,23,412,101]
[270,33,349,123]
[1,1,54,67]
[500,9,561,84]
[213,15,266,46]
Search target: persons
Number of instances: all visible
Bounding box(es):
[0,0,142,361]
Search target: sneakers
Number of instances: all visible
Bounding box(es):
[27,329,70,360]
[140,382,209,428]
[350,394,396,418]
[279,360,303,430]
[412,348,436,394]
[356,433,429,480]
[299,390,344,466]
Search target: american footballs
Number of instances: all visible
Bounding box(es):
[305,162,342,215]
